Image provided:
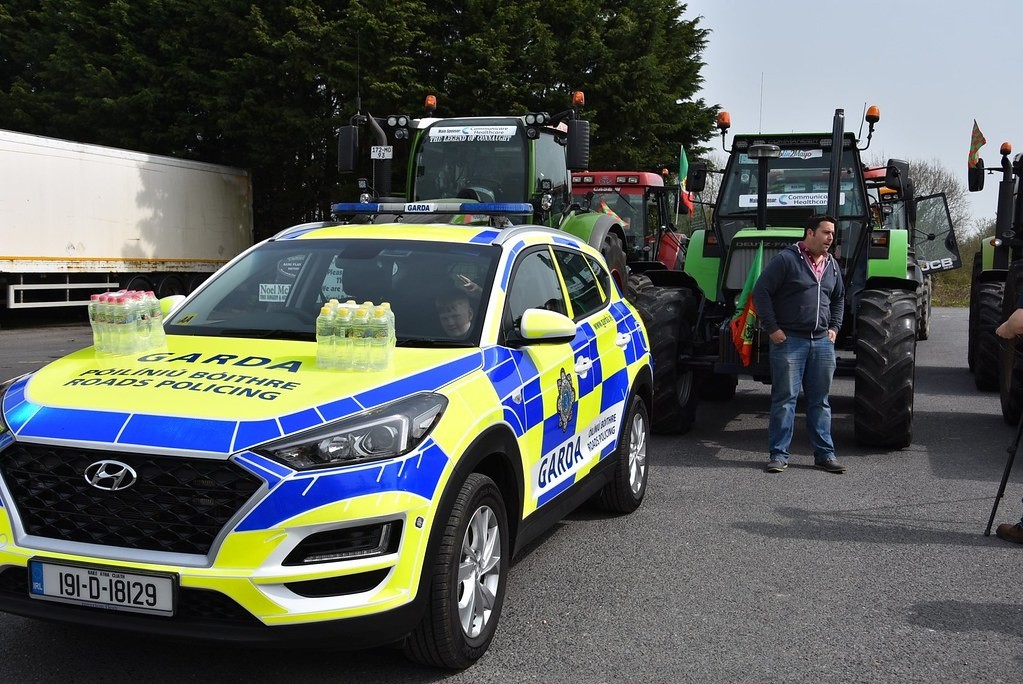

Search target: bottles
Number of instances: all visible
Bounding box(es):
[316,299,396,371]
[88,290,166,355]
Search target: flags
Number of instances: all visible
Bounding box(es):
[968,120,987,168]
[728,243,763,366]
[679,149,694,219]
[598,201,629,228]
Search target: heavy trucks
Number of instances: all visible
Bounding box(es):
[0,129,254,327]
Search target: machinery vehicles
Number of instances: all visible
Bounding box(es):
[337,30,689,298]
[635,71,961,453]
[967,119,1023,424]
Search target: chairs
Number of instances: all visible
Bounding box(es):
[343,262,383,296]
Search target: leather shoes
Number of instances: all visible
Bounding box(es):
[996,524,1023,543]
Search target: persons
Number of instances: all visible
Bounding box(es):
[436,274,483,337]
[335,245,385,297]
[751,215,845,473]
[996,295,1023,545]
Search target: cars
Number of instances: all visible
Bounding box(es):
[0,201,651,667]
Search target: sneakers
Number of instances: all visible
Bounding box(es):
[815,459,847,471]
[766,458,788,471]
